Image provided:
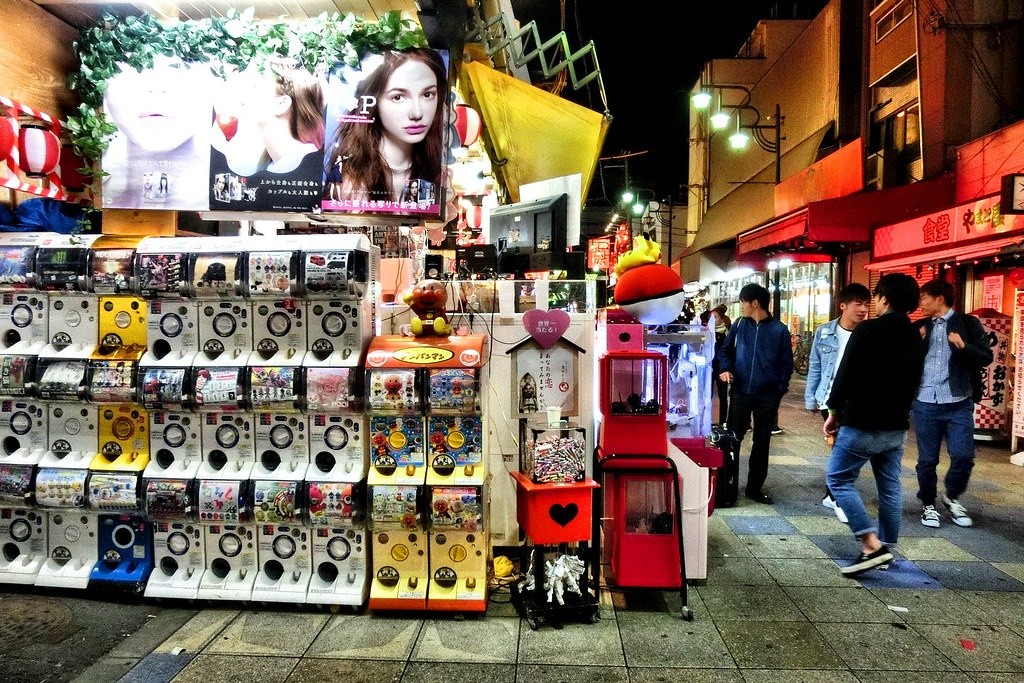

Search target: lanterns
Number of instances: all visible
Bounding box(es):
[0,107,14,162]
[17,124,60,179]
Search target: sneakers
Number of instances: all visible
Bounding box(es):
[874,560,889,571]
[942,493,973,528]
[922,504,941,527]
[821,494,834,508]
[841,545,893,574]
[832,500,850,523]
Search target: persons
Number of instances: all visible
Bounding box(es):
[209,63,324,211]
[913,279,994,527]
[803,282,871,523]
[103,55,210,211]
[823,272,925,575]
[701,283,795,504]
[326,49,446,214]
[457,219,473,247]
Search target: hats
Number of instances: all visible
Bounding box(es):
[712,303,727,319]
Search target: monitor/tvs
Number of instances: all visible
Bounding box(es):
[489,193,568,279]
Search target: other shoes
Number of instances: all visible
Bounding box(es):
[747,426,752,431]
[771,426,783,435]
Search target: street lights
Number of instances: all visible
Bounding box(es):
[622,189,675,269]
[691,84,786,322]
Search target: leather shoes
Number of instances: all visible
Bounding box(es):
[744,486,774,504]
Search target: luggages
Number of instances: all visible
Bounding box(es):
[711,422,740,507]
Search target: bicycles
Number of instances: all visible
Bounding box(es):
[791,333,815,375]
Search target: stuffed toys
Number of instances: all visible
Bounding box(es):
[615,231,662,279]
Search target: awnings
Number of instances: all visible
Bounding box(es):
[466,60,614,213]
[736,172,957,272]
[680,119,835,259]
